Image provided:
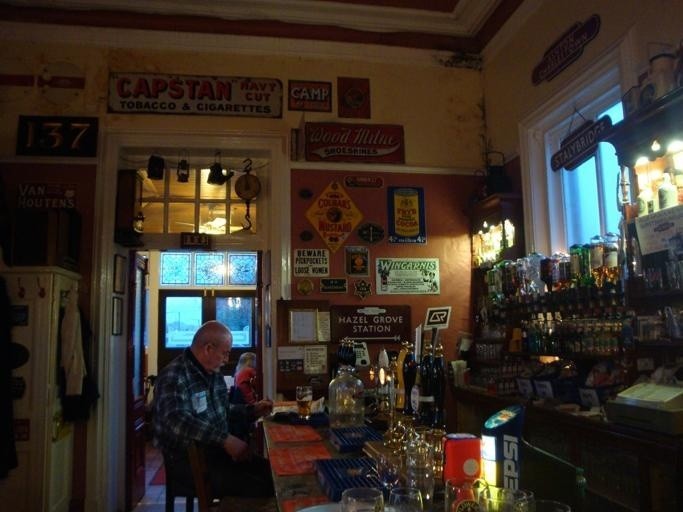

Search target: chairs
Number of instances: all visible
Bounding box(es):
[186,444,279,511]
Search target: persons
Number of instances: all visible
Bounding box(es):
[235,352,257,405]
[144,375,155,412]
[149,320,275,497]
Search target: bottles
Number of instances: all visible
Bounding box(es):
[447,218,683,397]
[381,270,387,291]
[637,167,678,217]
[328,337,447,428]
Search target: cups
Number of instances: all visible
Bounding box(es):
[296,385,313,419]
[337,410,572,512]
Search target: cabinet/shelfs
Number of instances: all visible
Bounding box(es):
[1,266,82,512]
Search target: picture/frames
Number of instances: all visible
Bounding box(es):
[113,254,127,295]
[112,297,123,336]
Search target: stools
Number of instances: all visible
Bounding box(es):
[166,485,197,511]
